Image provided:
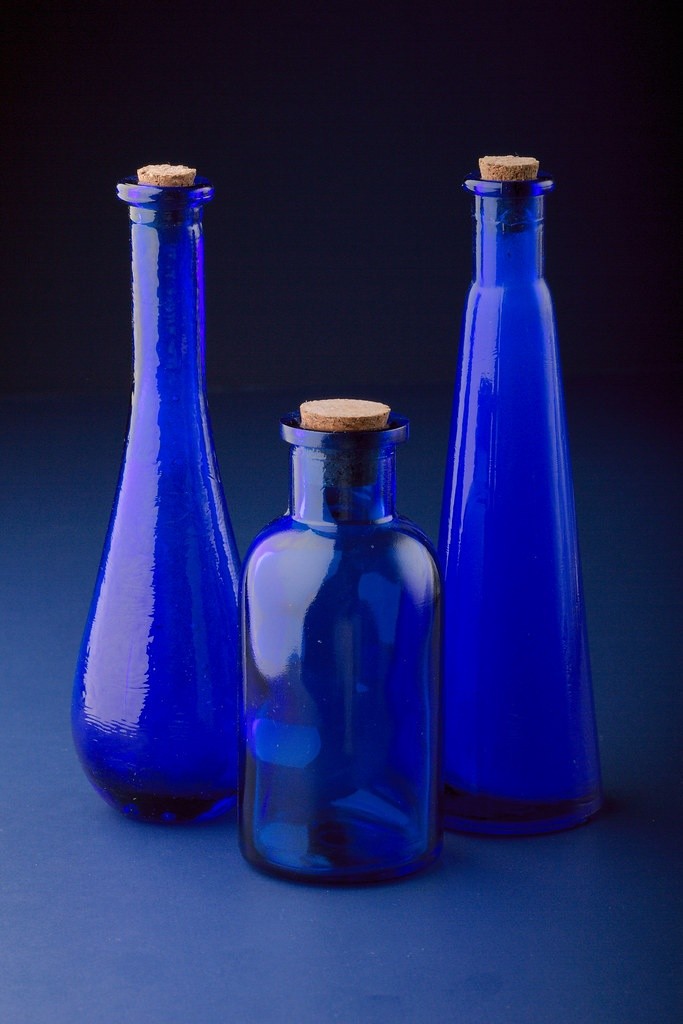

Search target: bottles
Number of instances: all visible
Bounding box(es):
[72,165,244,830]
[443,157,605,834]
[242,402,441,887]
